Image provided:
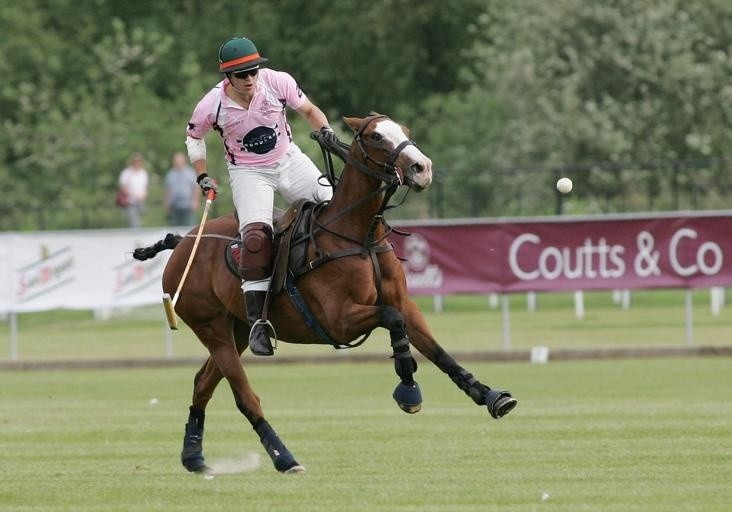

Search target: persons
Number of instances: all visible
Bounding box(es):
[115,152,150,228]
[160,150,201,227]
[185,36,340,354]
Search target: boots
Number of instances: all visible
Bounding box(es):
[243,286,275,357]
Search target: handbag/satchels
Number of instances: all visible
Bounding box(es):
[114,188,131,210]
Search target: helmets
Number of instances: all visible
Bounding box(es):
[218,35,269,74]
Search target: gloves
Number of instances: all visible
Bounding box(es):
[318,126,339,145]
[196,173,219,200]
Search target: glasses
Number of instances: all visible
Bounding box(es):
[228,68,258,81]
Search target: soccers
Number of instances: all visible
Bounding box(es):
[557,178,572,193]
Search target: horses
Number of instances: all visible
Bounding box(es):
[133,111,518,476]
